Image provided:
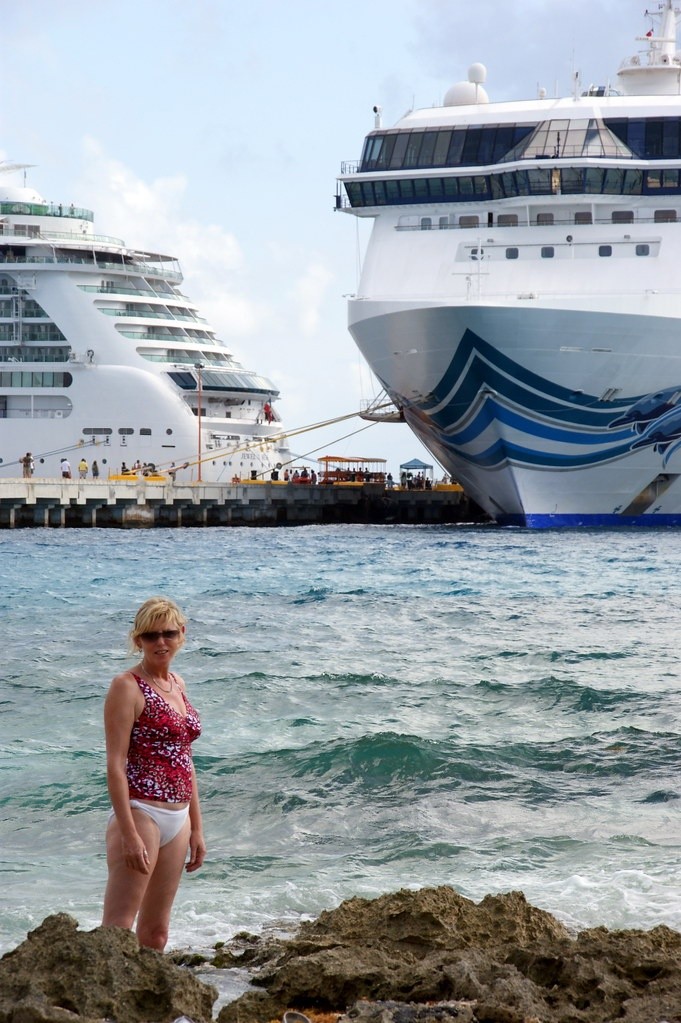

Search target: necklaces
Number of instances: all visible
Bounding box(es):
[141,662,172,693]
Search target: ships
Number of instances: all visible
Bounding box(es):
[331,0,681,527]
[0,163,291,483]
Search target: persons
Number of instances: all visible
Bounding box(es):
[58,204,63,216]
[132,460,149,476]
[61,459,71,479]
[284,468,310,481]
[78,458,88,479]
[400,471,432,490]
[101,597,205,950]
[70,203,74,215]
[121,462,130,475]
[19,452,35,478]
[335,467,369,482]
[169,462,176,481]
[442,473,457,484]
[387,473,393,488]
[311,469,316,484]
[92,461,99,479]
[271,469,279,481]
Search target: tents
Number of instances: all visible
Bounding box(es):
[399,458,433,489]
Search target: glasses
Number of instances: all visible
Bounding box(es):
[139,630,181,642]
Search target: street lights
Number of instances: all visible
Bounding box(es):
[194,362,204,482]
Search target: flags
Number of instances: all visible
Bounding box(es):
[646,29,653,37]
[263,397,272,425]
